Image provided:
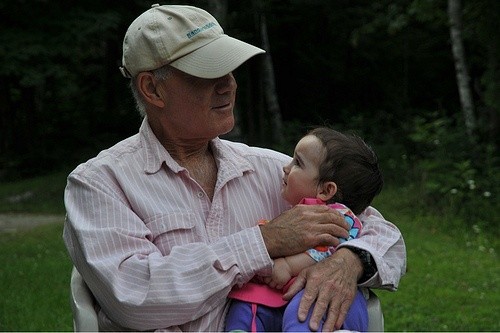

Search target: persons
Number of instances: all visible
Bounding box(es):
[62,3,408,332]
[224,128,407,332]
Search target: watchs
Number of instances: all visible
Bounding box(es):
[346,246,377,285]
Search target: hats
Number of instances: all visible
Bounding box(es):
[119,3,266,79]
